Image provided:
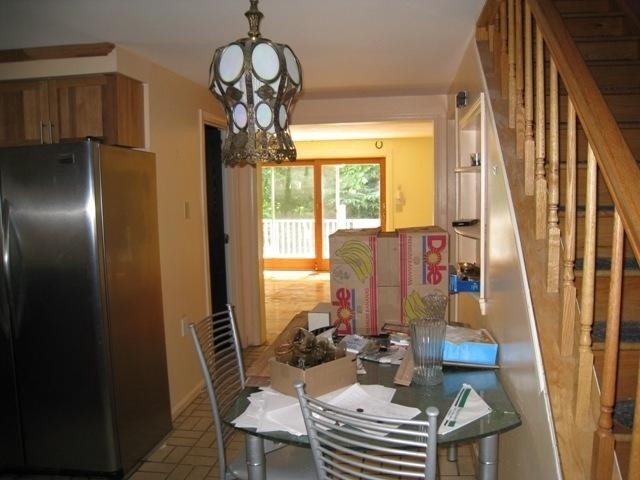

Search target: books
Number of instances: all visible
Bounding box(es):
[228,382,422,441]
[425,334,503,370]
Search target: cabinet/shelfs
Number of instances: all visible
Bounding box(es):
[0,73,149,150]
[455,92,486,314]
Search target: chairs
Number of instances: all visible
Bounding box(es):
[186,309,331,480]
[291,378,441,480]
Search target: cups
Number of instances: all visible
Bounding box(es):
[274,327,346,369]
[468,152,481,166]
[408,316,446,387]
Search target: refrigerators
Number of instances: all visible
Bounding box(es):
[0,144,174,479]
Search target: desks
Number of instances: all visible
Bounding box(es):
[222,299,522,479]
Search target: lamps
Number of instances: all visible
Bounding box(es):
[202,1,306,167]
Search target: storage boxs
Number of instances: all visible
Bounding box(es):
[417,325,499,365]
[324,227,449,335]
[266,350,358,398]
